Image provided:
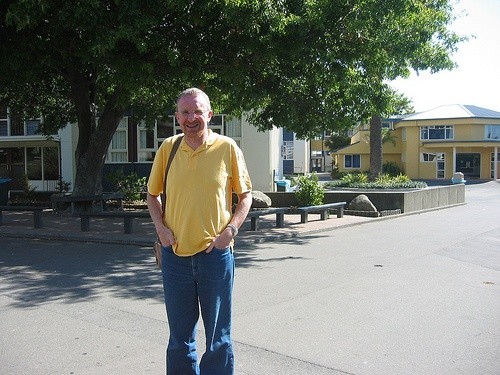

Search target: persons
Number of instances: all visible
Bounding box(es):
[147,87,252,375]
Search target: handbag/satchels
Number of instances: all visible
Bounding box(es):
[153,236,164,268]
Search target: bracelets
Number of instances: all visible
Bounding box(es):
[228,224,238,235]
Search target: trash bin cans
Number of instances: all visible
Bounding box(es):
[276,180,291,193]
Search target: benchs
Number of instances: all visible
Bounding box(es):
[0,205,44,228]
[79,212,154,236]
[245,208,289,230]
[50,192,124,214]
[296,202,347,222]
